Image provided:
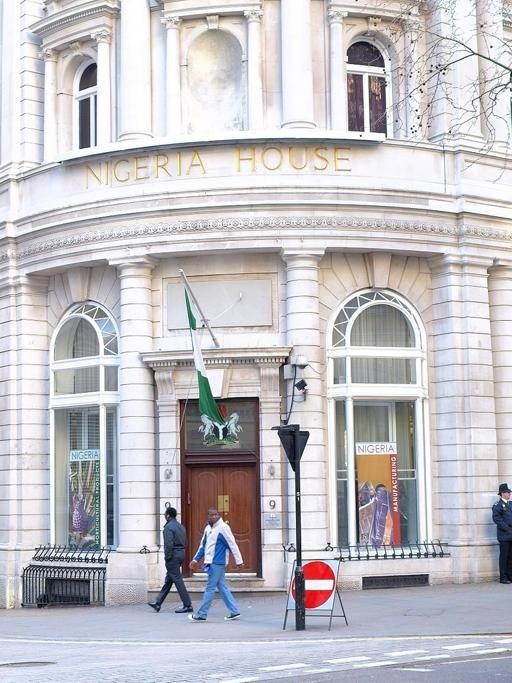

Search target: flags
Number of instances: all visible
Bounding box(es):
[185,287,226,425]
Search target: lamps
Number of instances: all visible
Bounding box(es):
[283,354,309,424]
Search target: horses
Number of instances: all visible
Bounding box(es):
[227,411,243,438]
[198,413,215,441]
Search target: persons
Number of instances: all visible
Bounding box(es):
[492,484,512,584]
[188,506,244,622]
[147,507,193,613]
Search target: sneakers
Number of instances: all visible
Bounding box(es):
[188,614,206,621]
[500,580,511,584]
[224,613,241,620]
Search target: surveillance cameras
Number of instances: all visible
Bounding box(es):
[294,380,307,391]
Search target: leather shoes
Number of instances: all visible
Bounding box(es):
[175,607,193,612]
[149,603,160,612]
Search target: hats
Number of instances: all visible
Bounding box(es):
[498,484,511,495]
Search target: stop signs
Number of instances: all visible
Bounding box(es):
[287,559,340,610]
